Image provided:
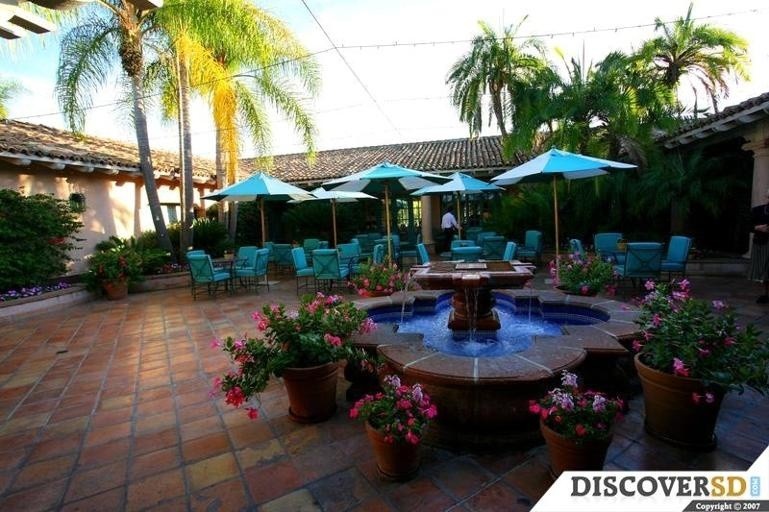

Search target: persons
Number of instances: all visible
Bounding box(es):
[441,208,464,252]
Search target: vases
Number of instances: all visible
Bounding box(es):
[540,416,615,481]
[633,352,729,449]
[365,420,422,481]
[280,361,339,423]
[102,272,128,300]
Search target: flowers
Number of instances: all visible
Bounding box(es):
[633,278,769,404]
[207,291,388,420]
[349,374,438,446]
[528,370,626,443]
[83,248,146,294]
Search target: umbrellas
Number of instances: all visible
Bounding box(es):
[199,170,318,280]
[409,171,509,243]
[287,184,381,245]
[321,161,453,268]
[490,144,640,284]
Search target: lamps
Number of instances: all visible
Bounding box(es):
[69,192,86,213]
